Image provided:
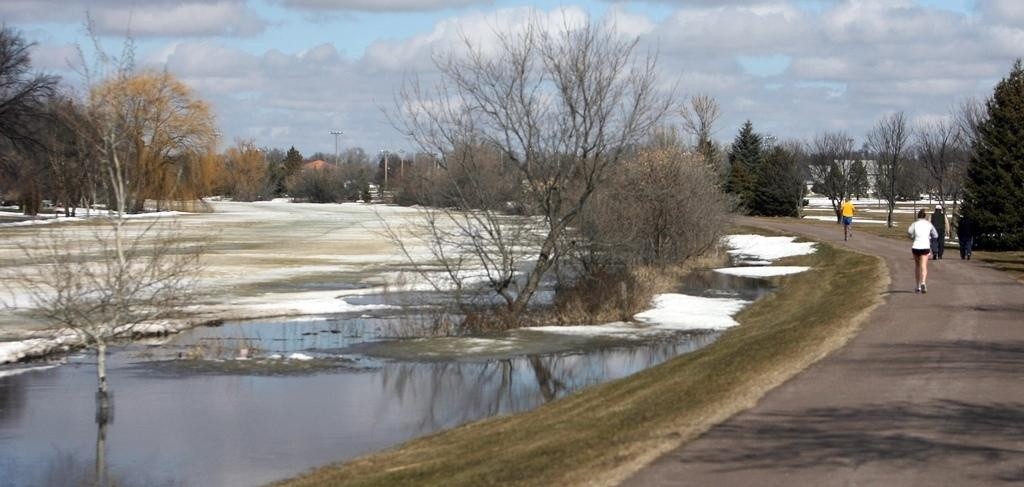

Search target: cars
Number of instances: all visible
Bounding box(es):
[36,199,68,214]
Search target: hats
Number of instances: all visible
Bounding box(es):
[936,205,942,209]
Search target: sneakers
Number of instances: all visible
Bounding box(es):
[961,257,965,259]
[850,233,852,237]
[938,256,942,259]
[921,283,927,293]
[933,257,936,259]
[916,288,921,292]
[845,238,848,240]
[968,253,971,260]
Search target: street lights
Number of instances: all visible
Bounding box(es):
[326,126,406,189]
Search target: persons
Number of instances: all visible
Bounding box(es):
[928,203,950,260]
[956,209,975,260]
[838,196,857,241]
[907,208,939,293]
